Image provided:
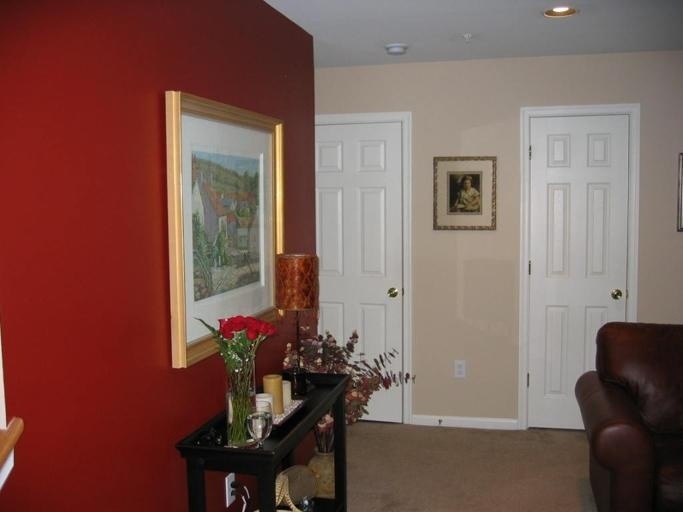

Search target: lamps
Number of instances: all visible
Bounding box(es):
[275,253,319,355]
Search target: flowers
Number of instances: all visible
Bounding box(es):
[283,330,417,453]
[194,314,276,444]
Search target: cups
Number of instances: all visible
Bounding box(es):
[283,381,293,408]
[262,374,282,413]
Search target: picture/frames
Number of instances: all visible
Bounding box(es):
[165,90,284,369]
[432,156,498,232]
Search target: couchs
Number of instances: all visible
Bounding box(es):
[576,322,683,511]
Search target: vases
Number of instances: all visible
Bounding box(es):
[309,447,335,499]
[228,364,256,449]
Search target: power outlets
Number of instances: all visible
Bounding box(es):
[225,473,236,507]
[454,360,467,382]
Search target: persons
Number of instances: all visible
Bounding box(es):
[454,175,479,212]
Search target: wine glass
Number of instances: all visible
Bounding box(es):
[246,401,273,449]
